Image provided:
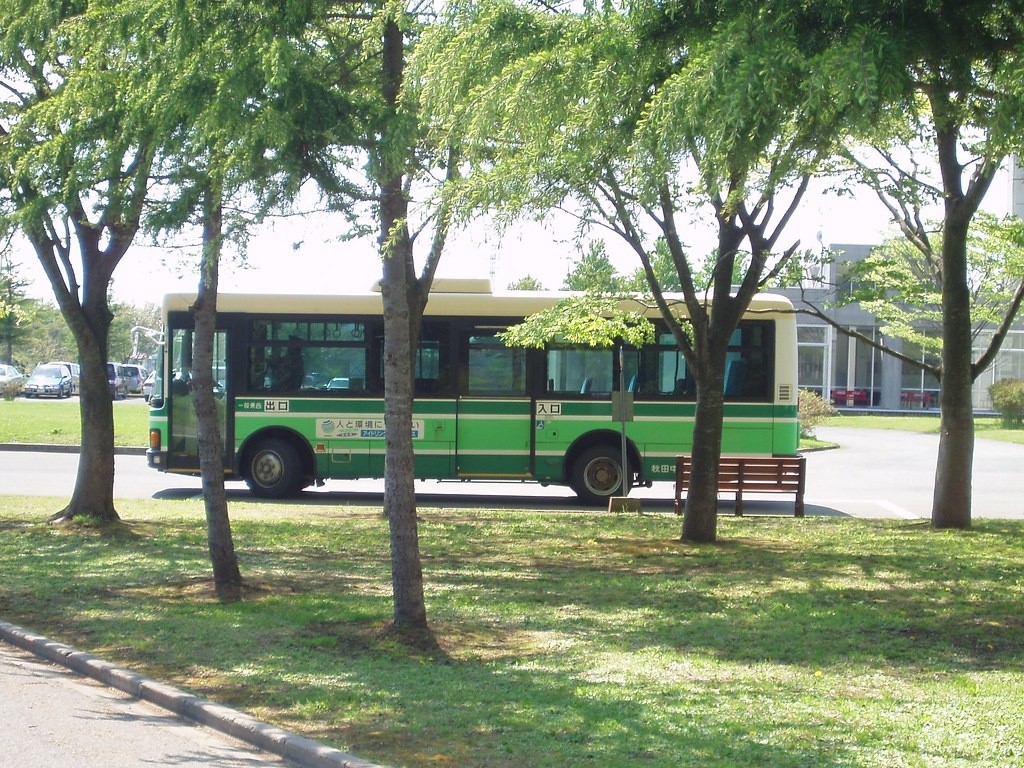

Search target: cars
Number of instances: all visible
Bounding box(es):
[1,358,156,404]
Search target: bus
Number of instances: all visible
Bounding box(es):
[135,275,801,507]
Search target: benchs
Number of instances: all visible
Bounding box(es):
[674,453,806,518]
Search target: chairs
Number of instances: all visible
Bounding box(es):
[580,359,748,398]
